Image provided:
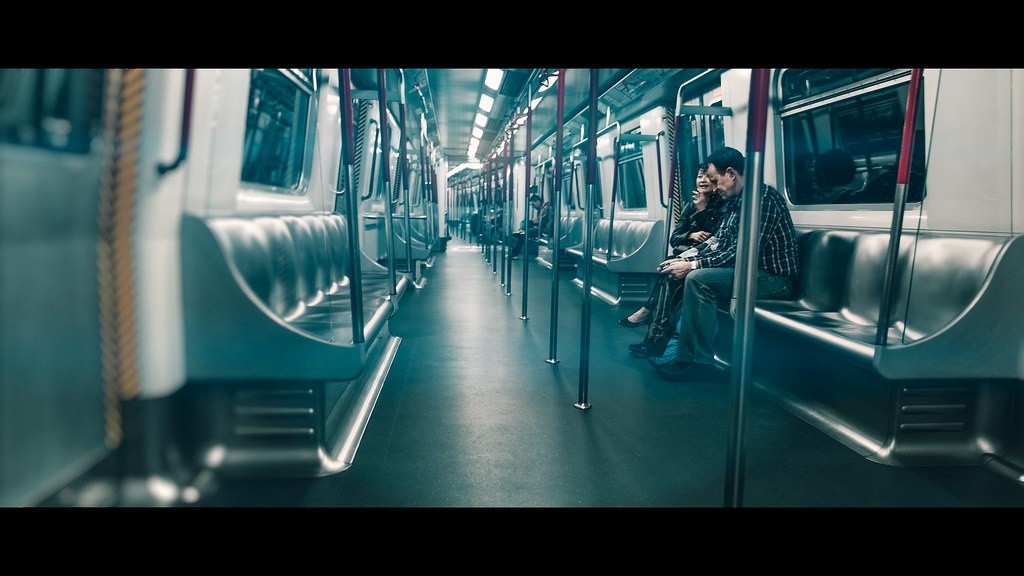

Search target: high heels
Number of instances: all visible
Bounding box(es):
[617,310,653,328]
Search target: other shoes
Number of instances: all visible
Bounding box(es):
[505,254,519,260]
[656,358,720,381]
[629,337,666,357]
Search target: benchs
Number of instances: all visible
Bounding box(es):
[566,219,665,304]
[683,227,1024,470]
[181,206,402,484]
[379,216,437,289]
[535,215,585,268]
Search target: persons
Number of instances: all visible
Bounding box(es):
[505,195,554,259]
[618,163,725,354]
[476,199,502,243]
[649,147,800,380]
[795,129,924,203]
